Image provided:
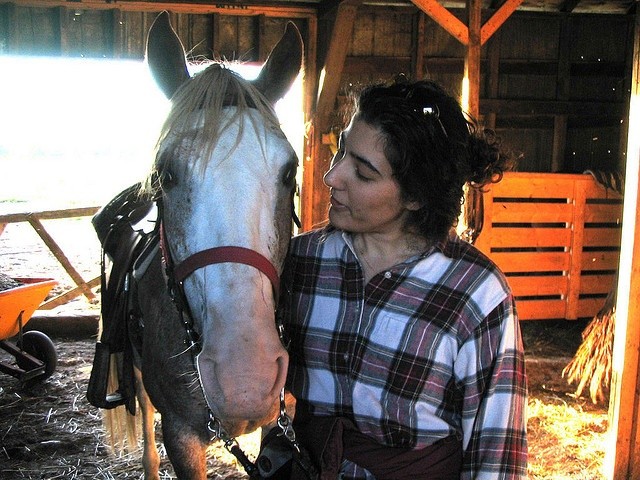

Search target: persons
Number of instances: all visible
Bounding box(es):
[269,75,529,480]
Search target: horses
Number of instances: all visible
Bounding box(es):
[97,12,303,479]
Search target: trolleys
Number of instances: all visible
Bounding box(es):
[0,276,59,387]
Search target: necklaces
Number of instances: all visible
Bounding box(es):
[357,235,424,277]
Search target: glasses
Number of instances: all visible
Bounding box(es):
[389,80,450,136]
